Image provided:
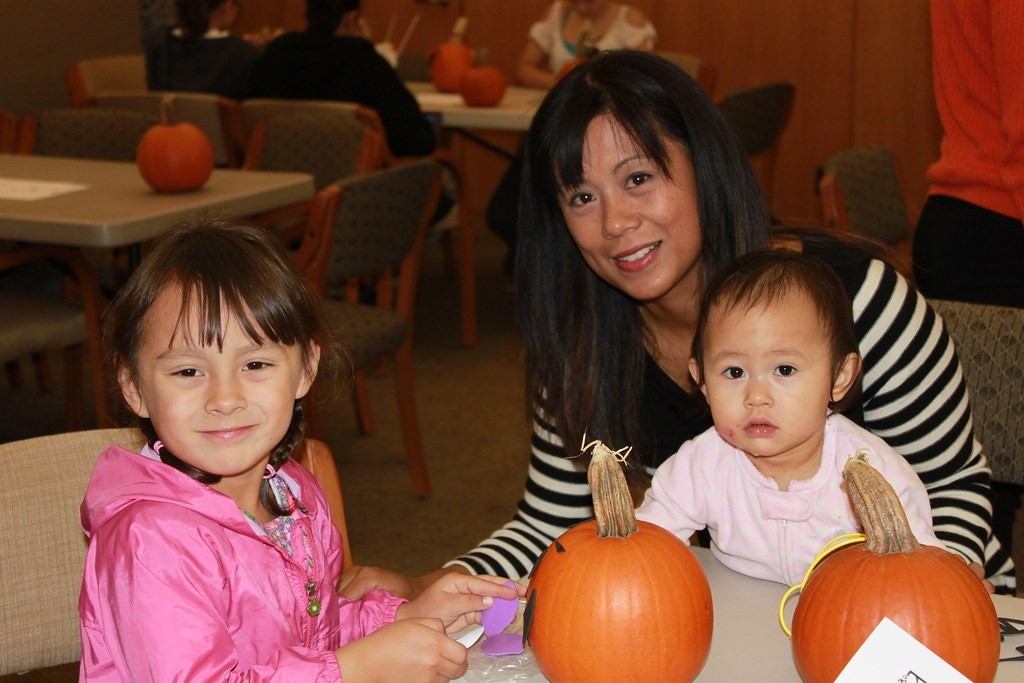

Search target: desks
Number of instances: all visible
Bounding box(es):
[430,522,1024,683]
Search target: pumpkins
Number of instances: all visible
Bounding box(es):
[790,450,1000,681]
[524,424,712,681]
[136,93,212,191]
[428,17,474,93]
[461,46,506,105]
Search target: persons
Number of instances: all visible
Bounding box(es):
[622,243,939,594]
[486,0,660,275]
[329,45,1023,612]
[157,1,257,99]
[73,220,532,683]
[229,0,453,225]
[904,1,1022,569]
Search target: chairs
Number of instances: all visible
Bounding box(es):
[708,81,796,213]
[65,52,153,100]
[272,150,452,501]
[232,98,439,299]
[228,118,380,251]
[8,105,160,309]
[812,147,923,268]
[0,239,129,435]
[149,91,243,169]
